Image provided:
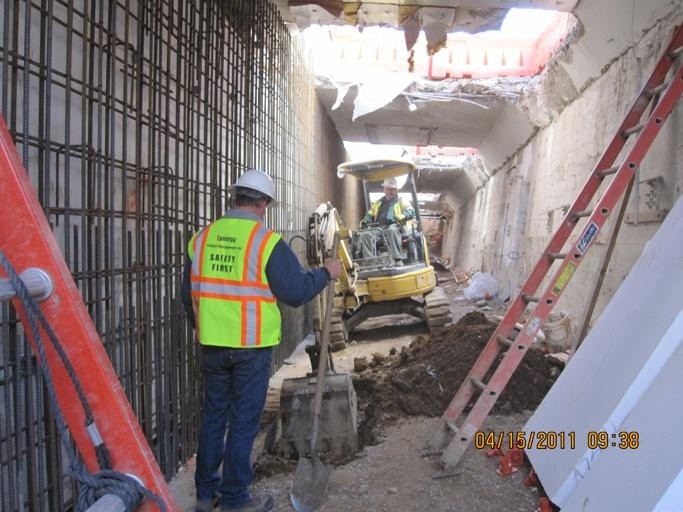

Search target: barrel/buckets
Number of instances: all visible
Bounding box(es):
[541,311,575,354]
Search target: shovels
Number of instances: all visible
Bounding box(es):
[290,234,340,512]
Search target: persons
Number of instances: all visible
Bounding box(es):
[358,175,417,270]
[181,168,346,512]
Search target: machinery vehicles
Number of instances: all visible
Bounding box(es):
[279,158,454,461]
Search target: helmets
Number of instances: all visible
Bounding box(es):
[227,169,276,200]
[380,176,399,188]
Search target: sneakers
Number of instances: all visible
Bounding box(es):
[393,258,404,267]
[222,494,272,511]
[194,496,222,512]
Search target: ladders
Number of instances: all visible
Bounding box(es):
[428,19,683,469]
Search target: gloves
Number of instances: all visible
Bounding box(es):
[359,219,367,228]
[394,214,405,221]
